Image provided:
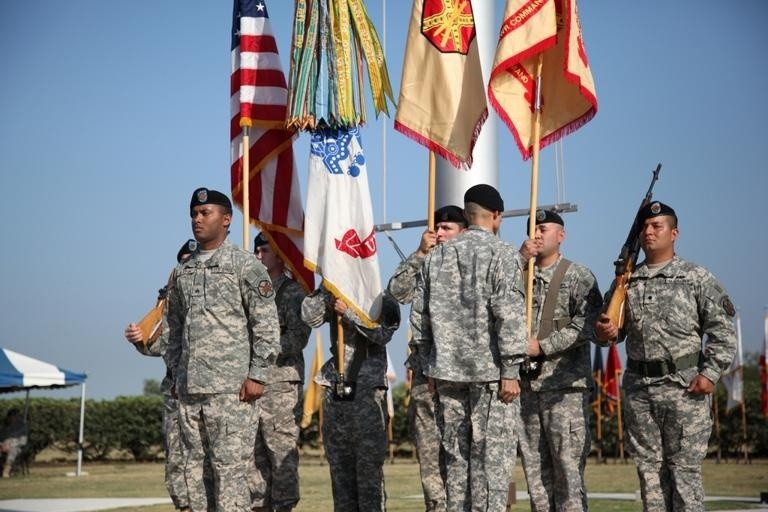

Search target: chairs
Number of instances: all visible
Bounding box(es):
[0,433,36,475]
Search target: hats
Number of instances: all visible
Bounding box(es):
[190,188,232,210]
[254,232,270,247]
[527,209,564,236]
[434,205,464,227]
[177,239,200,262]
[464,183,504,212]
[639,201,674,225]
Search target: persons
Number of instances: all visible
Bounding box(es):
[300,270,401,512]
[0,407,28,478]
[247,229,314,512]
[389,208,465,512]
[519,209,603,512]
[595,200,736,512]
[162,188,280,512]
[125,237,215,512]
[409,184,528,512]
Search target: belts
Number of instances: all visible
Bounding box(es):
[626,350,702,377]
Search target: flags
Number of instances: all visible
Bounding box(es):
[394,1,490,169]
[488,1,599,160]
[303,126,382,332]
[231,1,316,298]
[720,316,745,414]
[404,325,414,407]
[591,345,607,420]
[301,336,326,428]
[756,316,767,420]
[383,347,396,419]
[602,345,621,422]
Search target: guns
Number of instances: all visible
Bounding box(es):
[604,164,662,343]
[137,229,233,348]
